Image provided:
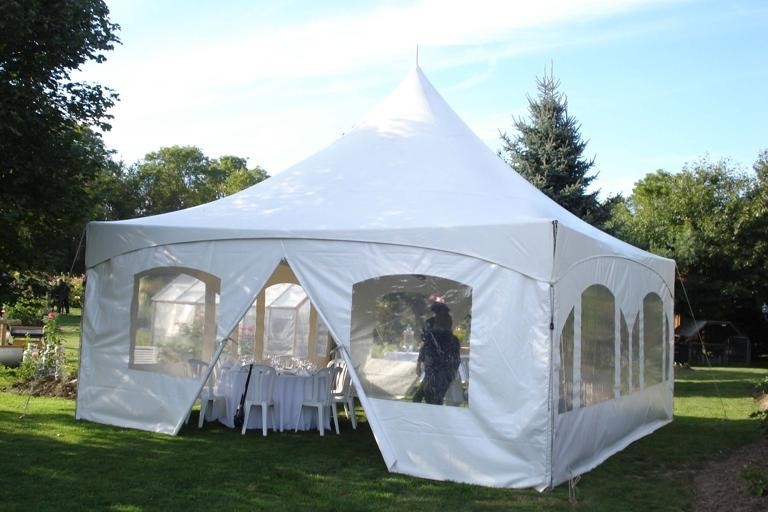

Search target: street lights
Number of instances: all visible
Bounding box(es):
[760,302,767,322]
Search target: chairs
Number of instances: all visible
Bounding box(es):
[183,353,360,437]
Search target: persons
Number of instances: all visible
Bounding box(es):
[412,301,462,405]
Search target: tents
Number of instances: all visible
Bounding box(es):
[76,60,680,494]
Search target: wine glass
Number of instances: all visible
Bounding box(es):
[240,355,317,377]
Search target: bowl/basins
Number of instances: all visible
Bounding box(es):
[384,351,419,363]
[459,346,470,355]
[0,345,24,366]
